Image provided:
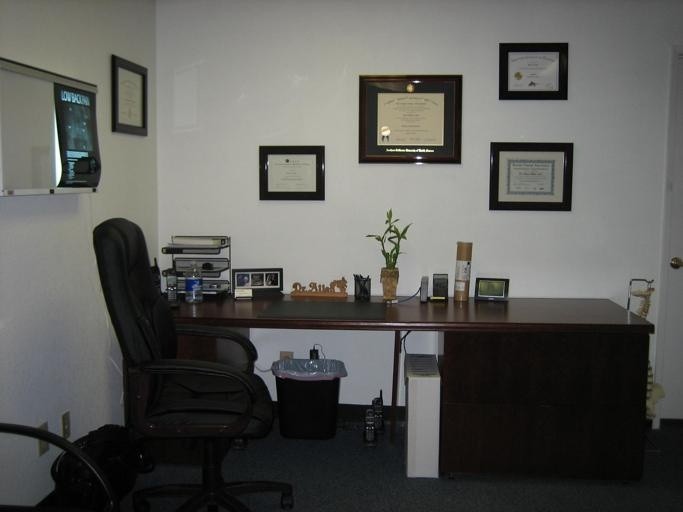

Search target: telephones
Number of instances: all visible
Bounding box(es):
[161,269,180,308]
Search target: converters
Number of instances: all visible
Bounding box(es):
[310,346,318,362]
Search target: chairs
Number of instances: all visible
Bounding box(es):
[92,216,297,512]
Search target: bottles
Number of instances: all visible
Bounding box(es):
[185,263,203,304]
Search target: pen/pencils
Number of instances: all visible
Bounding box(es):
[354,274,370,296]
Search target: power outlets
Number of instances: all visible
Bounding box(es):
[37,422,52,454]
[60,411,77,438]
[280,352,295,361]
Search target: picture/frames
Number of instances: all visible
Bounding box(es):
[232,269,286,290]
[488,141,572,211]
[111,55,151,137]
[475,278,510,300]
[258,146,327,201]
[498,42,568,101]
[359,74,463,163]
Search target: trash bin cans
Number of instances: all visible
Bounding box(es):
[271,357,348,440]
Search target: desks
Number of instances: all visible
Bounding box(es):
[159,294,655,485]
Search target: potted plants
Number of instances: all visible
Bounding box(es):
[367,210,414,303]
